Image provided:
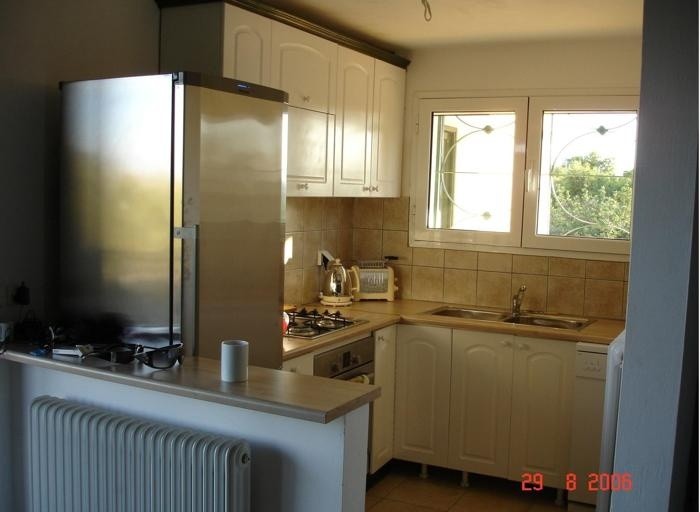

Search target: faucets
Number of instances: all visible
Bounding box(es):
[511,284,527,317]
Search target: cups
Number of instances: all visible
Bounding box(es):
[0,321,9,342]
[221,339,249,385]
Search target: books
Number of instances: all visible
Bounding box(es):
[51,345,81,357]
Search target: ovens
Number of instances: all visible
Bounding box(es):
[313,337,374,476]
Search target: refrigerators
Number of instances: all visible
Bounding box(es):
[55,69,288,371]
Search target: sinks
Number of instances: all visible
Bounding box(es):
[414,304,509,322]
[503,312,599,333]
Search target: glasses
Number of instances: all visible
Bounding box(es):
[85,343,186,368]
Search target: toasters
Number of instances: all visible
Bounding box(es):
[347,259,400,304]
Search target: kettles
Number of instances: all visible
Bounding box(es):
[320,256,361,307]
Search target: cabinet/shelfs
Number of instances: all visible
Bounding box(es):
[394,322,451,467]
[451,328,577,490]
[334,45,406,199]
[371,323,395,474]
[281,352,314,376]
[157,2,272,87]
[273,19,339,199]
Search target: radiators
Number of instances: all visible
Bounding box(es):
[27,394,253,511]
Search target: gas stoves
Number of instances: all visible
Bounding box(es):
[282,308,371,342]
